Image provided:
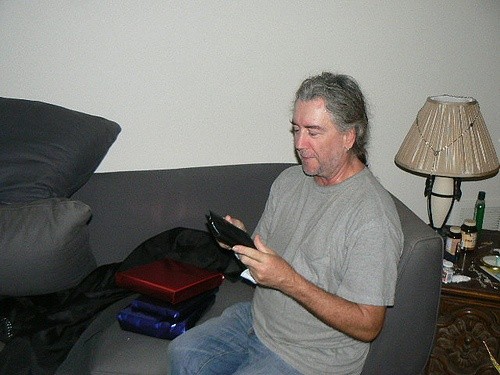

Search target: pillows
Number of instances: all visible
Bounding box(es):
[0,198,97,297]
[0,96,123,201]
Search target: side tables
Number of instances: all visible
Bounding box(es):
[422,225,500,375]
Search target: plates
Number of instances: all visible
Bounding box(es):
[483,256,500,267]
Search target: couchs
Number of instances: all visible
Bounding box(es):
[0,163,445,375]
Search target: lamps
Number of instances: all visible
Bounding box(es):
[394,94,500,232]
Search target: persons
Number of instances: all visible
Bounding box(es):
[166,72,405,375]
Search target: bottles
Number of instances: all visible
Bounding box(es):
[442,261,454,284]
[473,191,486,233]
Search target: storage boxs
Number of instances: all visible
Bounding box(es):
[114,258,224,341]
